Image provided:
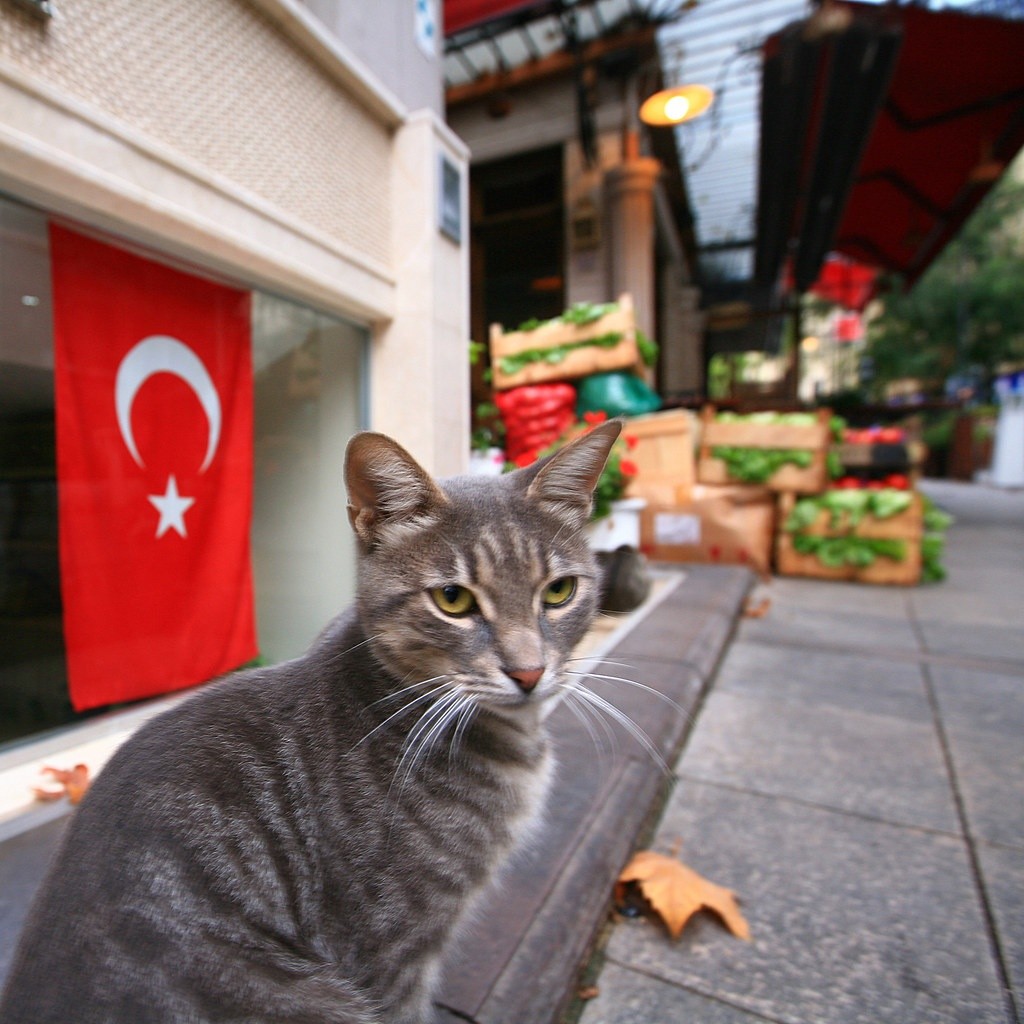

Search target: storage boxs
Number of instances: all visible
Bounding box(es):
[490,302,921,588]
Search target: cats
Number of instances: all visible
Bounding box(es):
[1,414,626,1024]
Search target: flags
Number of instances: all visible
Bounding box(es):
[47,222,260,713]
